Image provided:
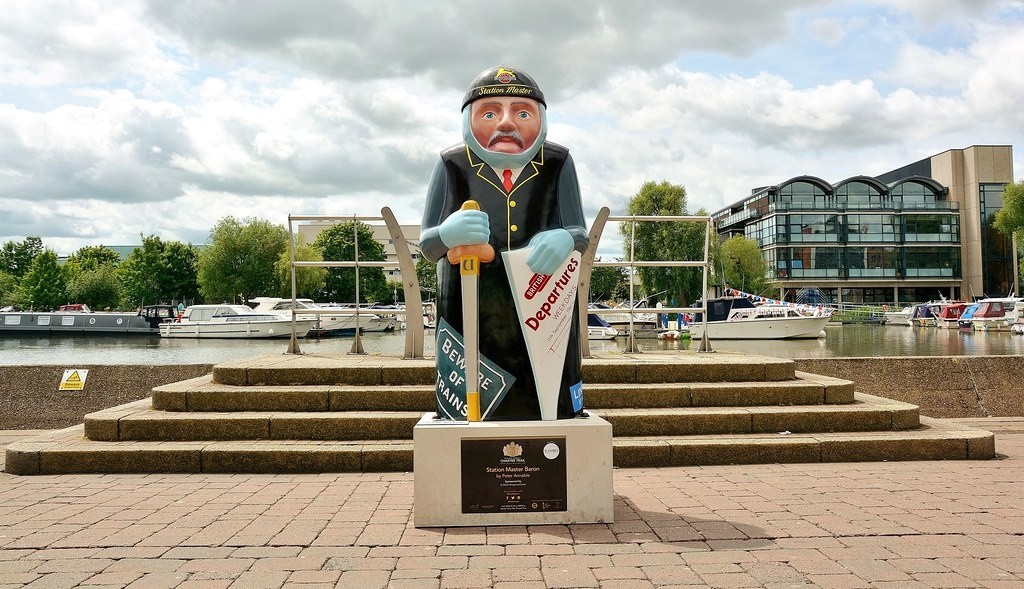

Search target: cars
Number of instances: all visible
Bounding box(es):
[58,303,91,312]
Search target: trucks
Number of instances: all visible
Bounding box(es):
[136,305,189,328]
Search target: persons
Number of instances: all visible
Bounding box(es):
[420,66,590,419]
[688,313,692,322]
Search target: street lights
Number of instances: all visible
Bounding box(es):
[29,300,35,312]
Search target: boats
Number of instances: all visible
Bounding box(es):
[586,298,657,325]
[686,296,831,341]
[587,314,620,341]
[250,297,438,333]
[158,303,322,339]
[885,297,1024,336]
